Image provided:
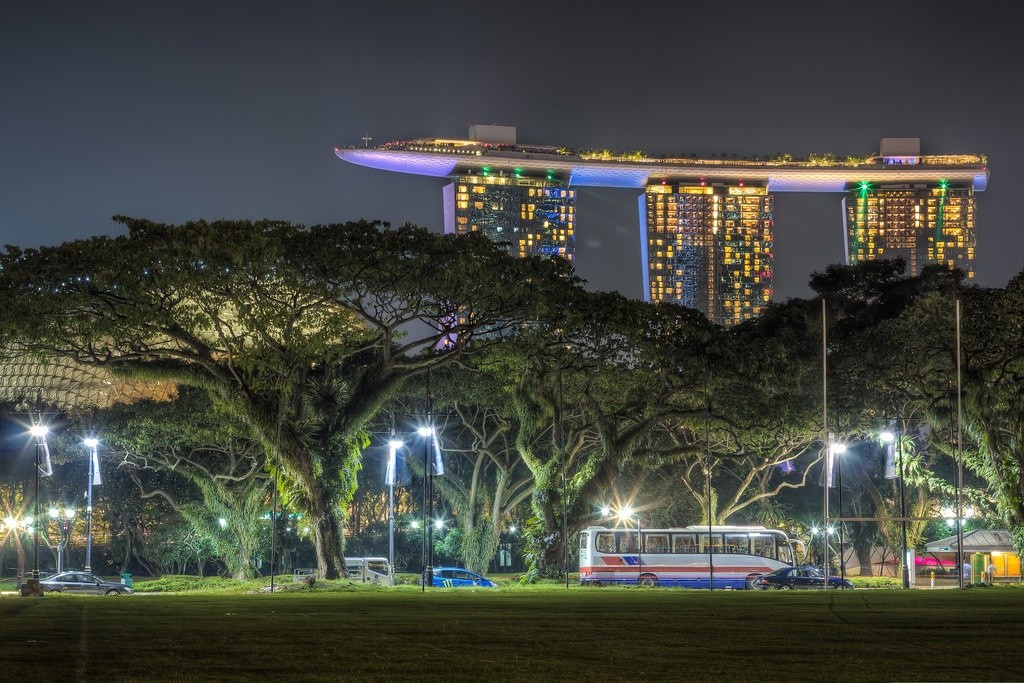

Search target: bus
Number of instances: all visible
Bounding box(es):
[579,526,806,591]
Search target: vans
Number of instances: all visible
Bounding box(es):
[343,557,391,587]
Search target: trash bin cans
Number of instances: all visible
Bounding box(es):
[120,572,133,587]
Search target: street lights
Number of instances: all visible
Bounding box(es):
[29,393,48,578]
[387,422,400,582]
[831,413,845,589]
[82,424,99,579]
[417,364,435,592]
[880,429,910,589]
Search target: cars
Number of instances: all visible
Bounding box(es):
[422,568,499,589]
[38,571,133,596]
[751,566,854,591]
